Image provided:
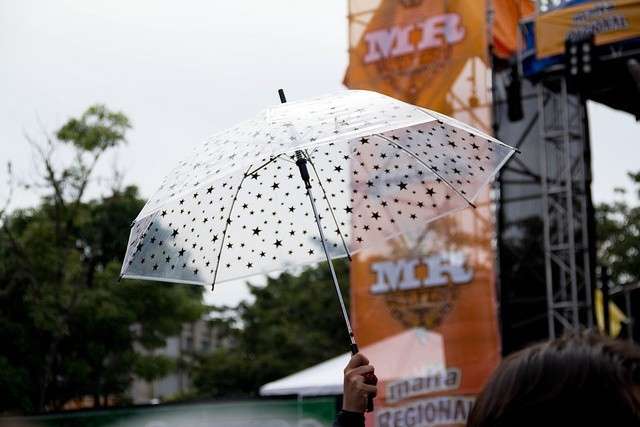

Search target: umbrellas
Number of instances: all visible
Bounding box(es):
[117,88,522,411]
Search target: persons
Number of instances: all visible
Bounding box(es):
[332,324,639,425]
